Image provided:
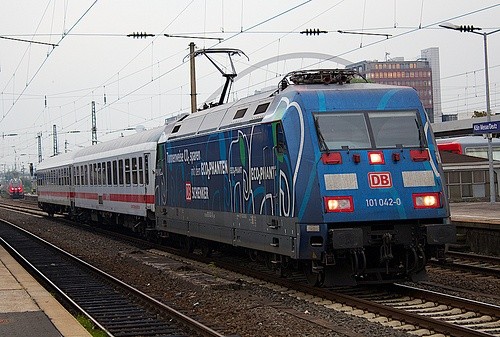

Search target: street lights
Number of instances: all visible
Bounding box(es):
[438,22,499,204]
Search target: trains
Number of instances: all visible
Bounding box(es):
[36,68,451,287]
[7,178,25,200]
